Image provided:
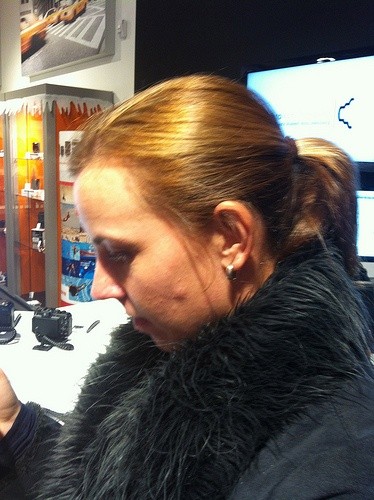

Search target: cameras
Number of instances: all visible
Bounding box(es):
[32,306,73,344]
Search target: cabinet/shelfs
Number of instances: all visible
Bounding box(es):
[0,83,115,311]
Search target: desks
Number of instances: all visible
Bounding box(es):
[0,297,132,427]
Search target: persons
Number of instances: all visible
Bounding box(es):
[0,74,374,500]
